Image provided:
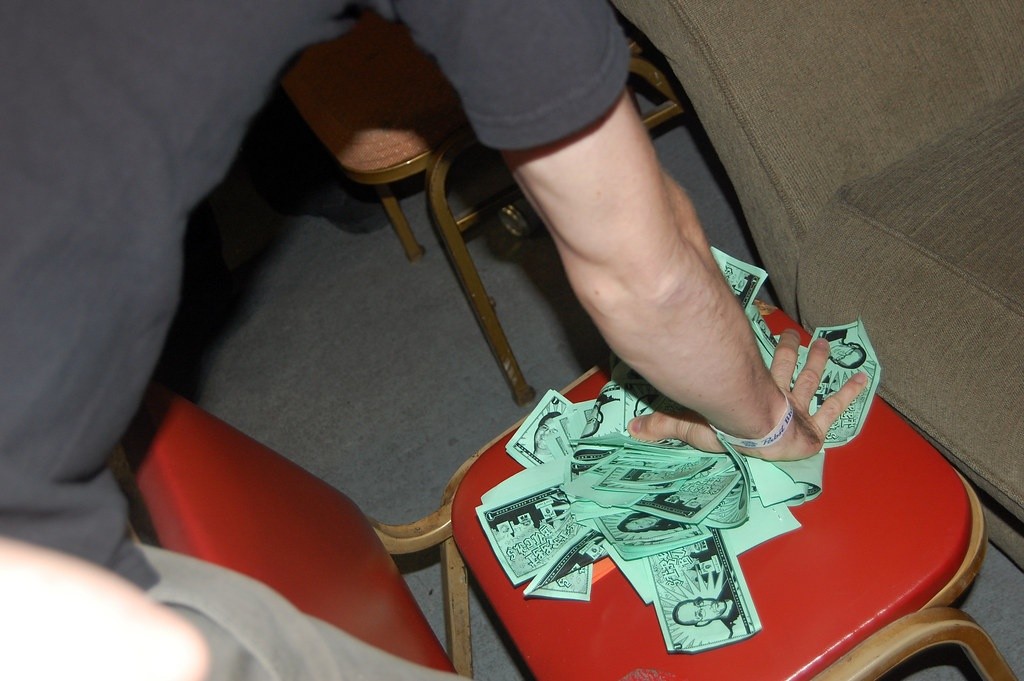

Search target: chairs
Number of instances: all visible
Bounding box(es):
[277,6,693,408]
[102,296,1019,680]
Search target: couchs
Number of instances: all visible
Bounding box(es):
[608,0,1023,572]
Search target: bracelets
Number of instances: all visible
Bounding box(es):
[708,392,793,448]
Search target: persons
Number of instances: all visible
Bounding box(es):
[1,0,867,680]
